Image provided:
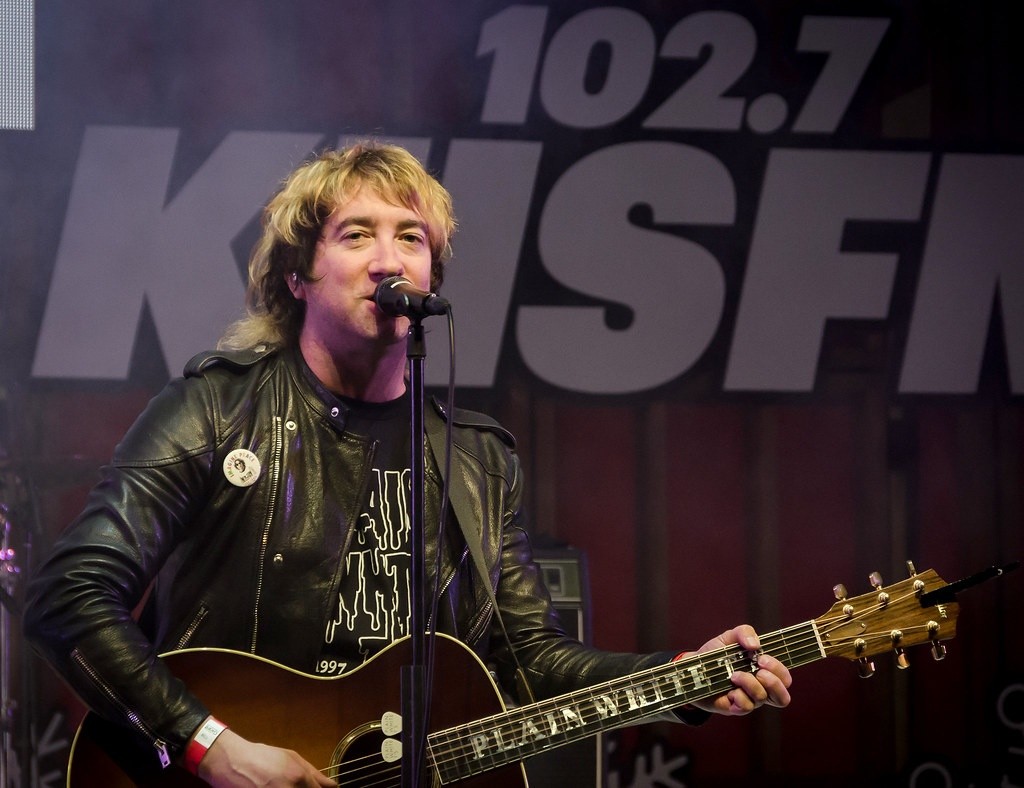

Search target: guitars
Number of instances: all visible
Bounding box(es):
[64,556,962,788]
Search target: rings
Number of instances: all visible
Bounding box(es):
[766,696,776,705]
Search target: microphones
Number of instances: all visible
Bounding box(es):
[374,276,451,318]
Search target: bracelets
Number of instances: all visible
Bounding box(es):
[185,715,230,776]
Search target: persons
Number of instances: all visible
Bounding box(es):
[20,141,795,788]
